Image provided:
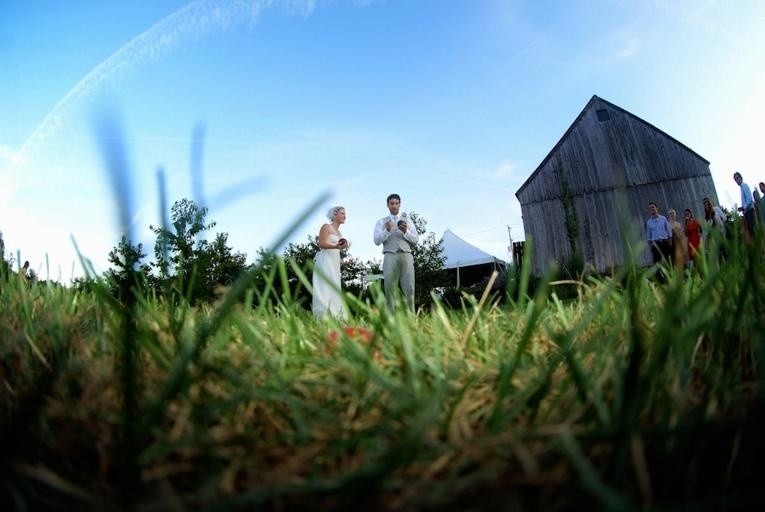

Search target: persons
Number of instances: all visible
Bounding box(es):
[758,182,765,227]
[310,206,353,321]
[645,202,673,285]
[373,194,419,318]
[683,209,702,266]
[703,201,726,240]
[666,207,686,283]
[702,198,732,240]
[733,172,756,240]
[753,190,761,223]
